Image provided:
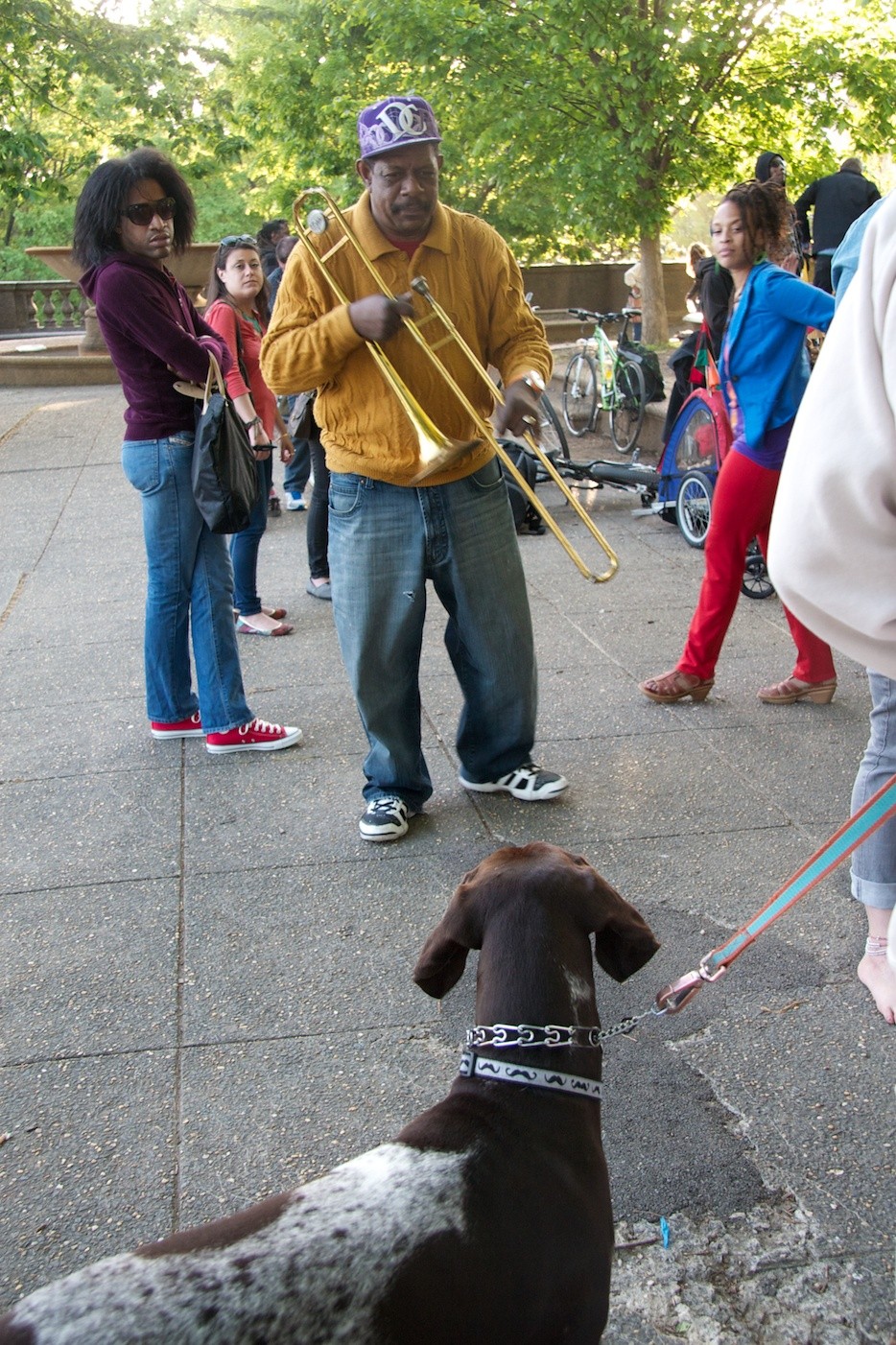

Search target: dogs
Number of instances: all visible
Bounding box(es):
[0,840,658,1345]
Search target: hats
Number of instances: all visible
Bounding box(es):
[359,98,444,159]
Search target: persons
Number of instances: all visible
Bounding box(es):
[260,97,569,842]
[768,188,896,1024]
[625,151,883,369]
[74,150,302,757]
[640,181,838,705]
[204,235,297,638]
[258,219,332,601]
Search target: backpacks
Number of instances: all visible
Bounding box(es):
[499,441,545,536]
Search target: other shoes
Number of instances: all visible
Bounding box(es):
[232,608,287,624]
[307,576,332,599]
[236,613,293,635]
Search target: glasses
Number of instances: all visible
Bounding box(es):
[219,234,258,247]
[119,195,178,226]
[770,160,785,167]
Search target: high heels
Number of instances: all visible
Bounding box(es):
[757,674,838,704]
[638,667,714,702]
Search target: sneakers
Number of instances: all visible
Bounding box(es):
[285,492,308,511]
[266,497,281,517]
[358,796,417,843]
[458,761,569,800]
[206,717,303,754]
[150,709,207,739]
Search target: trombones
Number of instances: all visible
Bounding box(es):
[290,187,621,585]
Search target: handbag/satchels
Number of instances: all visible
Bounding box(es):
[627,285,642,323]
[192,349,255,536]
[617,332,666,404]
[290,393,315,442]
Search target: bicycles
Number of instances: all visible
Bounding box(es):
[561,308,646,454]
[497,377,660,510]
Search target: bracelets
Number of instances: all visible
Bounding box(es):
[277,433,289,441]
[245,416,261,430]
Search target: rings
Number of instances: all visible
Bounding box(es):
[290,452,294,456]
[521,414,536,426]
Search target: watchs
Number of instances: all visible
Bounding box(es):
[509,370,545,399]
